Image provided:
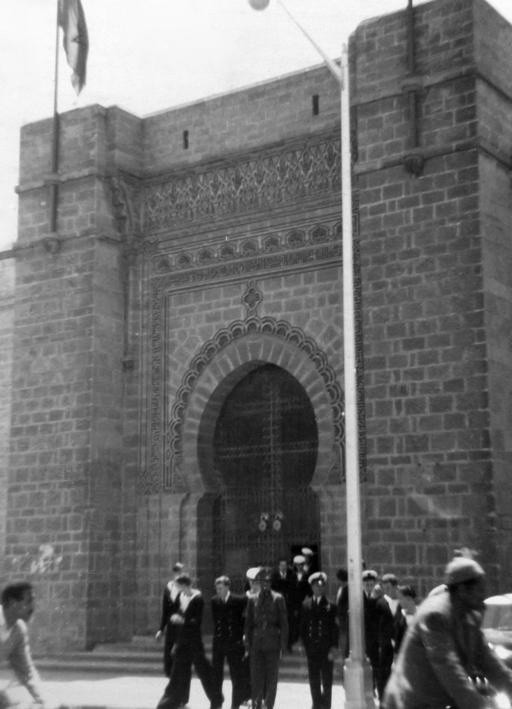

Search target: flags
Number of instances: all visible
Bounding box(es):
[56,0,89,96]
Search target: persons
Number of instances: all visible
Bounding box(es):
[0,579,46,708]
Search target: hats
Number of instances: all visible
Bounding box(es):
[293,556,306,565]
[300,547,316,558]
[362,569,378,581]
[307,571,328,585]
[244,567,260,580]
[257,567,275,582]
[445,555,487,585]
